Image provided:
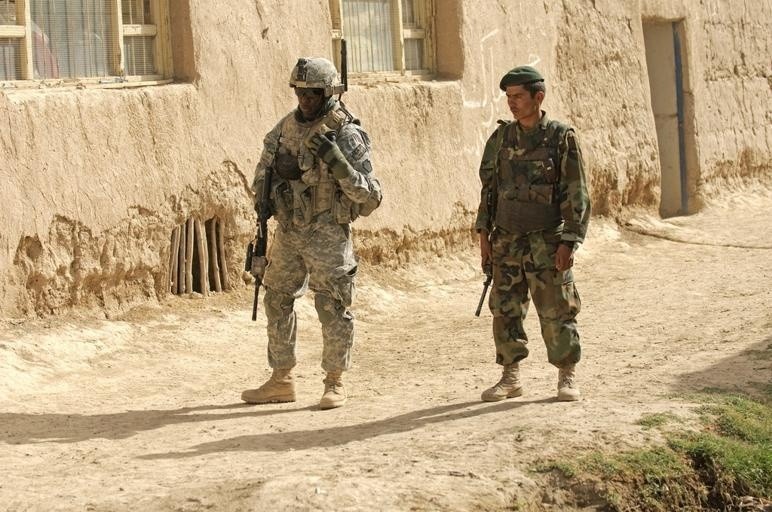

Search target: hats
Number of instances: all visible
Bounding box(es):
[500,66,544,91]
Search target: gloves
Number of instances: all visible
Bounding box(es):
[254,199,273,219]
[308,132,353,179]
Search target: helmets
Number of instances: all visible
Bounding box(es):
[289,57,337,89]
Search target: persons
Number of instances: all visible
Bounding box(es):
[475,67,591,401]
[241,57,382,409]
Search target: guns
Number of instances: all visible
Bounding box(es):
[245,166,273,322]
[472,184,496,317]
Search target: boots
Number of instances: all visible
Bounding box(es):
[558,363,579,401]
[481,362,522,402]
[241,369,296,404]
[320,371,347,409]
